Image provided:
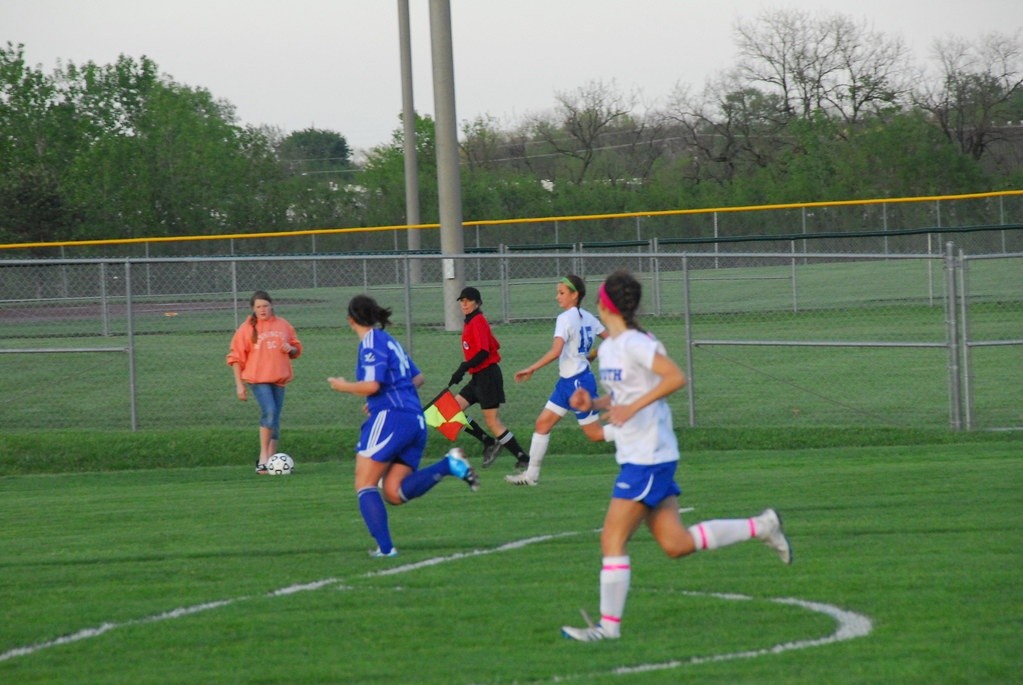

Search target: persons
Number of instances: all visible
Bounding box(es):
[561,271,793,644]
[227,290,302,476]
[503,274,609,486]
[448,287,530,468]
[327,294,481,558]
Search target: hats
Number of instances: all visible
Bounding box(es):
[457,288,480,300]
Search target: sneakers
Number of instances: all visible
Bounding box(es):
[368,546,398,557]
[515,461,529,468]
[480,438,502,468]
[561,610,609,643]
[758,508,792,565]
[504,471,538,486]
[445,448,479,492]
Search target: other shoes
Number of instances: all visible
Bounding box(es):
[255,461,268,475]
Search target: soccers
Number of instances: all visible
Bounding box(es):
[267,453,295,476]
